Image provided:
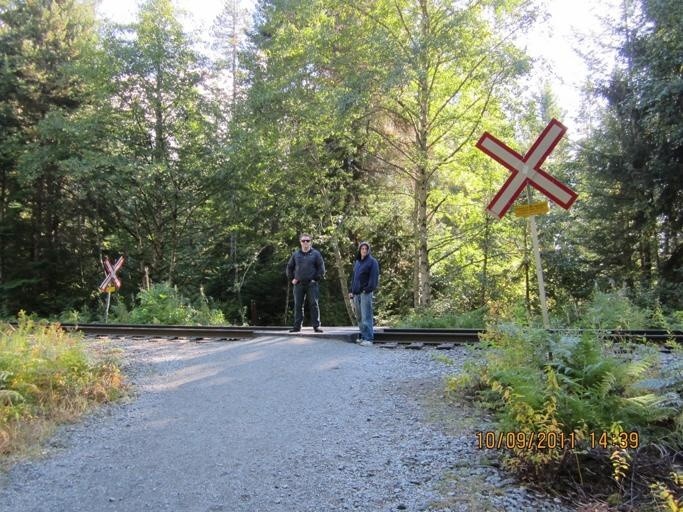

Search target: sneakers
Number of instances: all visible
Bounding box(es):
[356,338,373,346]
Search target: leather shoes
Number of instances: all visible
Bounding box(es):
[314,327,323,332]
[290,328,300,332]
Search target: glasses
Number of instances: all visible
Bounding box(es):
[302,240,310,243]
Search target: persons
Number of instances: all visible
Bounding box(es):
[347,242,379,347]
[285,234,325,333]
